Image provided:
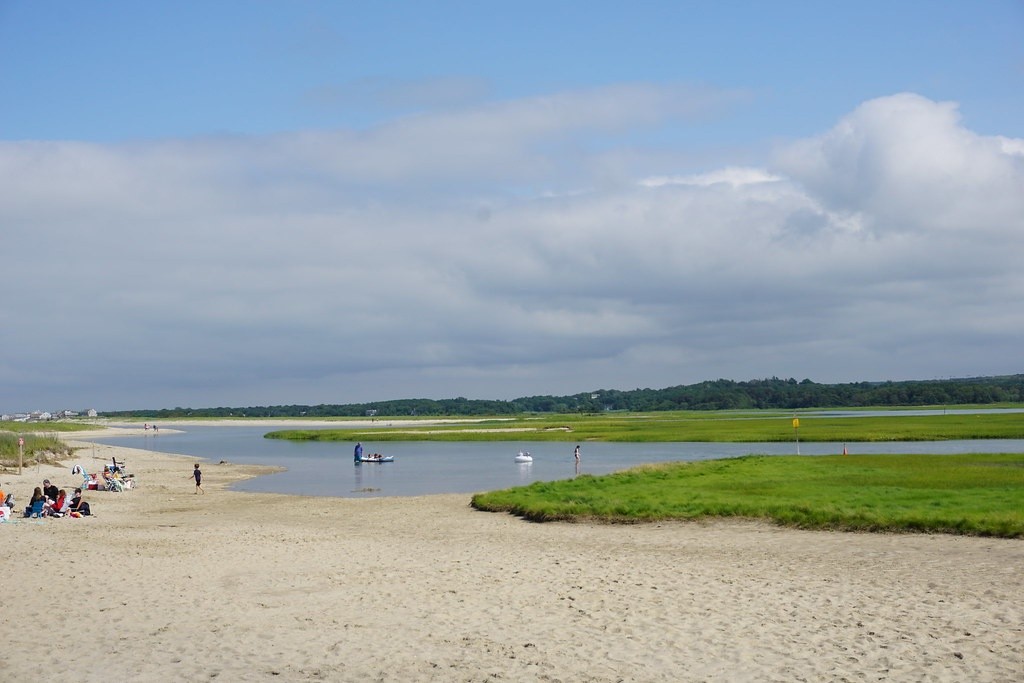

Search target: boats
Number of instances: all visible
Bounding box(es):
[514,450,534,462]
[360,455,394,462]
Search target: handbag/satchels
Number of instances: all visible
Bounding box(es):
[0,507,10,521]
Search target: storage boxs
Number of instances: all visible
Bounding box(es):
[88,484,104,490]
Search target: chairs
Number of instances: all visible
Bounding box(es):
[24,495,84,519]
[72,464,91,488]
[102,473,122,491]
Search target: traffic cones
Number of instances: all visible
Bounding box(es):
[843,443,847,456]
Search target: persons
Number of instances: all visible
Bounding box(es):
[188,463,205,495]
[0,483,4,507]
[103,467,124,485]
[353,441,362,462]
[518,450,523,455]
[29,479,82,517]
[368,453,383,460]
[574,444,581,467]
[144,423,150,431]
[153,425,159,432]
